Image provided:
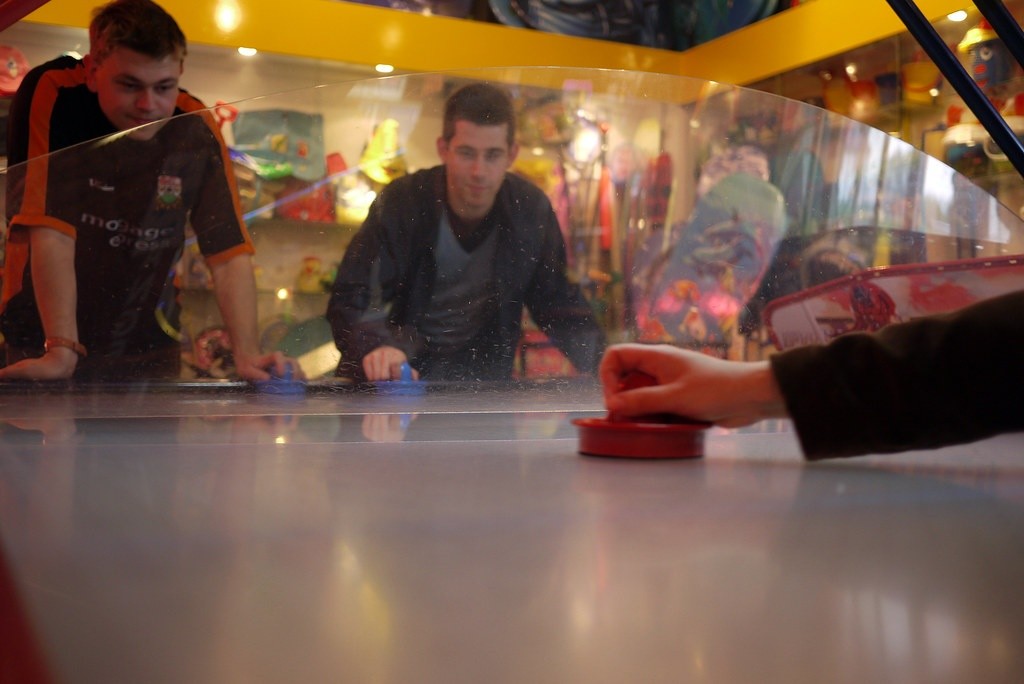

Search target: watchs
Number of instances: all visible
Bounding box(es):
[45,336,87,357]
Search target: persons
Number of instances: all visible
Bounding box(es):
[601,289,1024,462]
[0,0,301,384]
[326,83,608,381]
